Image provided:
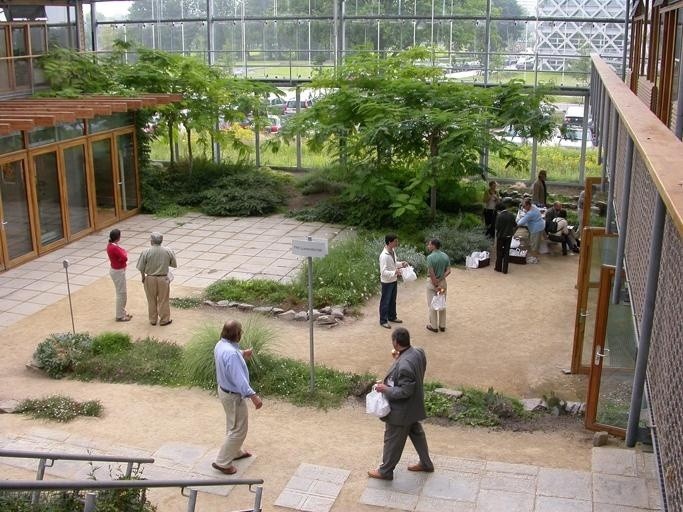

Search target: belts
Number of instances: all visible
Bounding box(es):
[220,386,241,394]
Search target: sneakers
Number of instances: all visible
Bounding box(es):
[526,256,540,264]
[126,314,133,317]
[160,319,172,326]
[115,317,130,321]
[572,245,579,253]
[575,239,580,247]
[150,321,156,325]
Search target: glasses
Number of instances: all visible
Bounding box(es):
[544,174,547,177]
[523,205,525,207]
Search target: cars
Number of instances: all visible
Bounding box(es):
[429,56,536,74]
[495,99,593,151]
[214,82,318,144]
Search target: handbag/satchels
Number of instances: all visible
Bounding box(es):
[509,249,529,258]
[431,291,447,311]
[509,236,521,248]
[465,256,479,268]
[399,260,416,284]
[471,251,490,262]
[365,385,391,419]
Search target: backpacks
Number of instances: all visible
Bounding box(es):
[549,218,564,233]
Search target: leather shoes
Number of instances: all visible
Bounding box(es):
[426,324,438,332]
[212,463,237,474]
[408,463,434,471]
[439,326,445,332]
[233,451,253,460]
[367,468,392,479]
[381,323,391,329]
[390,319,402,323]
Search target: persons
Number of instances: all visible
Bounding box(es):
[482,181,500,237]
[550,210,579,253]
[533,170,547,204]
[367,329,434,480]
[106,229,132,321]
[494,204,517,274]
[425,239,451,331]
[516,200,546,259]
[212,318,262,474]
[136,232,177,326]
[575,185,596,238]
[544,201,561,234]
[379,232,408,328]
